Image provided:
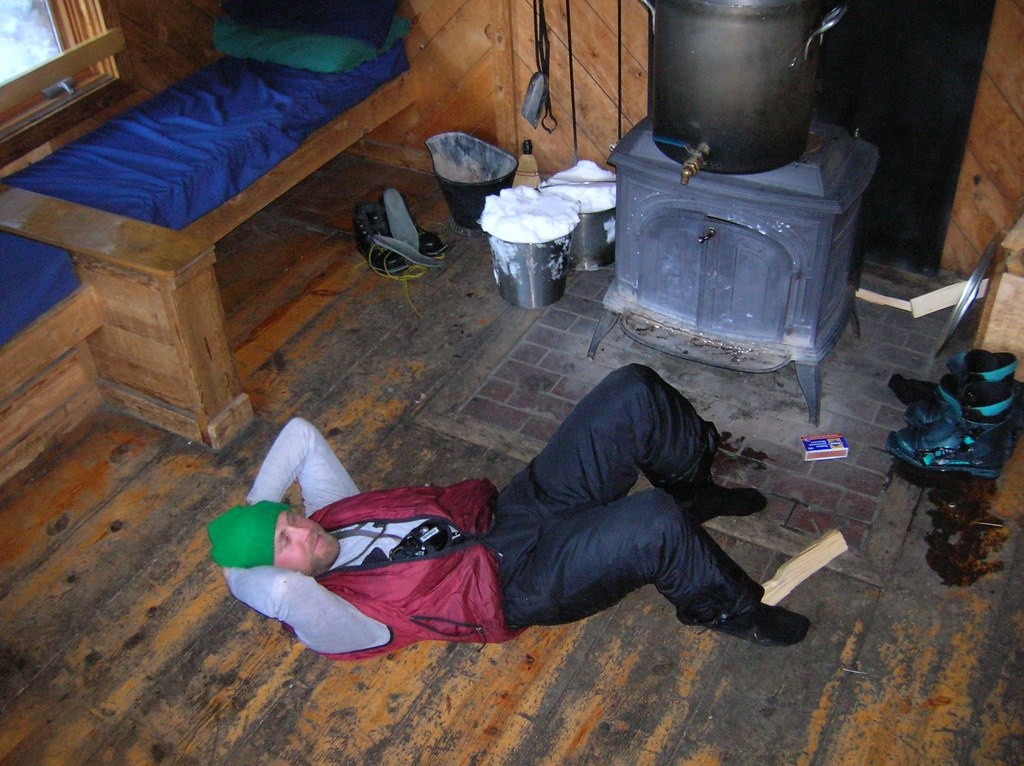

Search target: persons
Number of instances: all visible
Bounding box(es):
[206,362,811,660]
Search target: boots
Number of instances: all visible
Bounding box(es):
[885,374,1017,478]
[900,349,1018,427]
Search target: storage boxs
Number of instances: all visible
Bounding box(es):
[799,433,849,461]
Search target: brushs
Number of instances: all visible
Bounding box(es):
[511,140,542,189]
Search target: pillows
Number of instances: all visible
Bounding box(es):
[212,0,412,73]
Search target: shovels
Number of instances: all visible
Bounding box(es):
[520,0,549,130]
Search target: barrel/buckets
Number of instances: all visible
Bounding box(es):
[537,176,616,272]
[488,223,573,306]
[425,132,517,240]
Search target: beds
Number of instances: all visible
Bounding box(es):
[0,37,418,447]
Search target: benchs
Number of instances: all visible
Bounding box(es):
[0,226,102,486]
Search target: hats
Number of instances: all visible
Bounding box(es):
[207,500,292,568]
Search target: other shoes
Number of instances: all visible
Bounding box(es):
[379,192,446,255]
[352,202,408,274]
